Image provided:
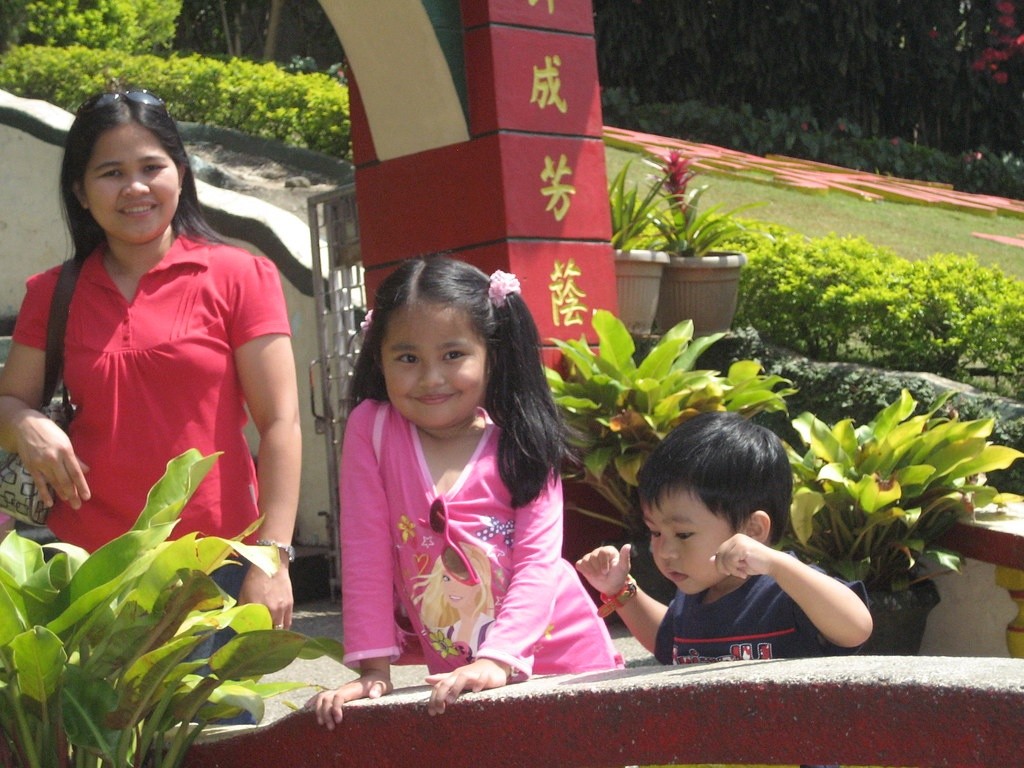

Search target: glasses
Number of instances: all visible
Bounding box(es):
[75,89,167,121]
[428,494,481,587]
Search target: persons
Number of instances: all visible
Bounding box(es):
[304,258,624,731]
[576,411,874,664]
[0,90,303,726]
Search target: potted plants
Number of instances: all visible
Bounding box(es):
[779,386,1023,654]
[641,145,774,338]
[608,157,672,337]
[540,308,799,607]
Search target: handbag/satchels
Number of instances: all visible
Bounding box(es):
[0,253,87,528]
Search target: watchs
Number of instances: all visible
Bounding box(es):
[255,540,296,563]
[597,574,637,618]
